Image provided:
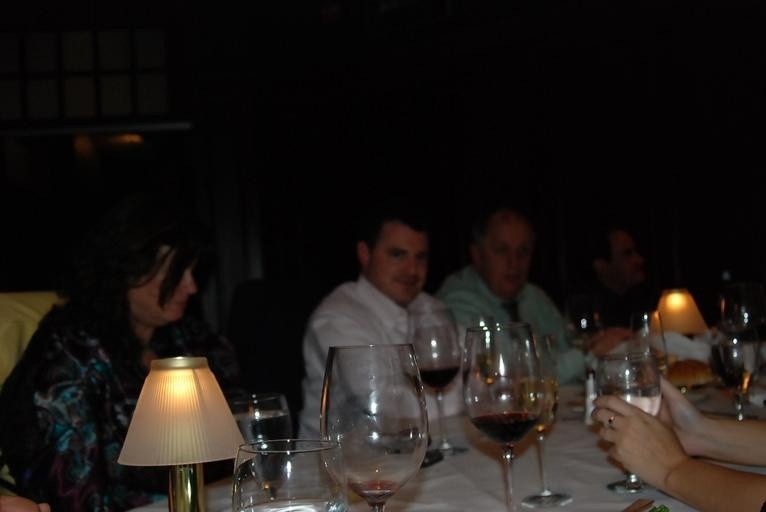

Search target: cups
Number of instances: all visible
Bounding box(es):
[234,390,292,500]
[319,342,429,511]
[233,438,348,512]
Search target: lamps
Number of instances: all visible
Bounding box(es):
[115,353,255,512]
[649,288,709,340]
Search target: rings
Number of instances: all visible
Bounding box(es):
[609,415,617,425]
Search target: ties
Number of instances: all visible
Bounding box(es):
[502,299,521,337]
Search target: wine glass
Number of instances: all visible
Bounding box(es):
[595,310,668,495]
[510,334,573,511]
[712,298,759,421]
[405,307,469,460]
[462,322,544,509]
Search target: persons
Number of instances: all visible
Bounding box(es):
[564,227,663,331]
[0,187,255,511]
[591,373,766,512]
[292,206,469,454]
[434,201,602,421]
[1,495,51,511]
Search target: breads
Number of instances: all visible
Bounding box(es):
[665,358,715,386]
